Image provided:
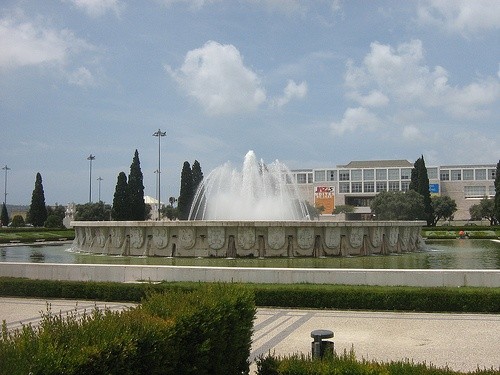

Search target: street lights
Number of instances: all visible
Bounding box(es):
[153,128,166,221]
[86,153,96,203]
[96,176,104,202]
[1,163,10,206]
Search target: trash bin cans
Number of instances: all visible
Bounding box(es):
[310,329,336,363]
